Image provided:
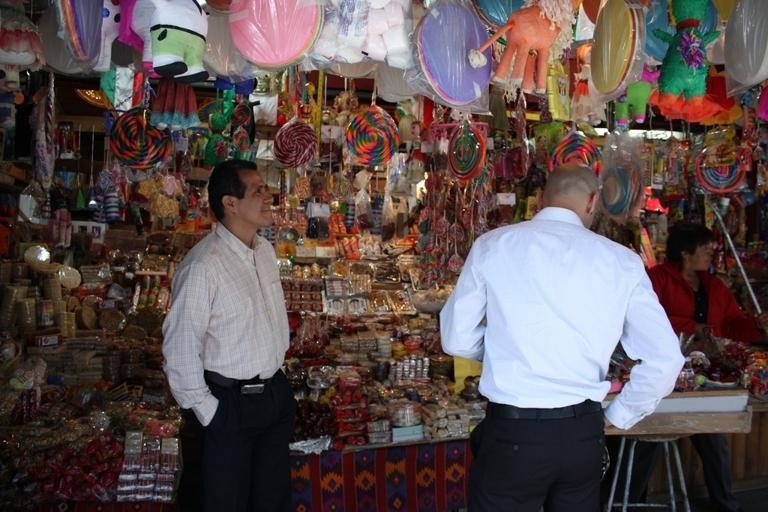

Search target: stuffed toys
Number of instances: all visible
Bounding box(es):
[651,1,721,105]
[118,0,165,80]
[570,43,607,126]
[469,1,565,96]
[150,1,209,86]
[89,0,120,72]
[614,68,661,133]
[312,1,415,70]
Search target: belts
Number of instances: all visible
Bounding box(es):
[486,400,601,420]
[204,373,267,396]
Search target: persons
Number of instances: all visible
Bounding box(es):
[439,164,686,511]
[621,221,768,512]
[161,159,297,512]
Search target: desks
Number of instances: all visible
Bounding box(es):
[602,386,753,511]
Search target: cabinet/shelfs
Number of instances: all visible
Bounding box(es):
[1,111,601,512]
